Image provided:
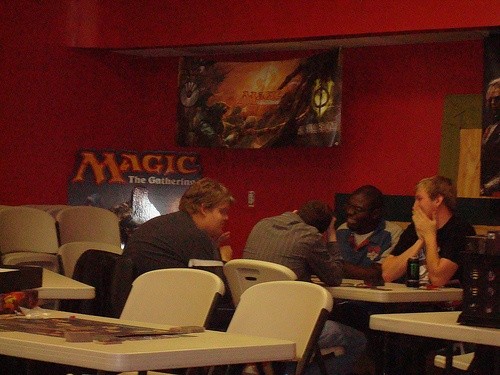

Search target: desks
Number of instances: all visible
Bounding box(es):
[307,273,500,375]
[0,263,96,310]
[0,307,297,375]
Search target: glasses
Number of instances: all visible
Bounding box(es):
[346,203,374,212]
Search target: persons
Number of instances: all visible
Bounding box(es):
[241,200,367,375]
[382,175,477,287]
[106,178,235,328]
[333,185,403,281]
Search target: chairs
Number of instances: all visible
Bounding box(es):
[0,202,333,375]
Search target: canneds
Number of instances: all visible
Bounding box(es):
[406,257,420,287]
